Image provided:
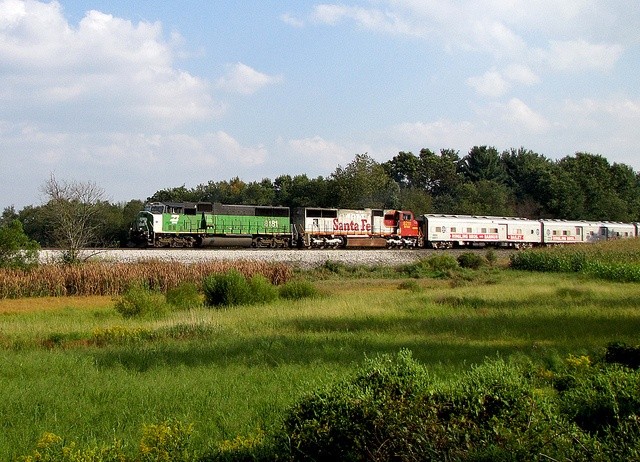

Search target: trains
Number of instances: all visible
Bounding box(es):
[126,198,640,251]
